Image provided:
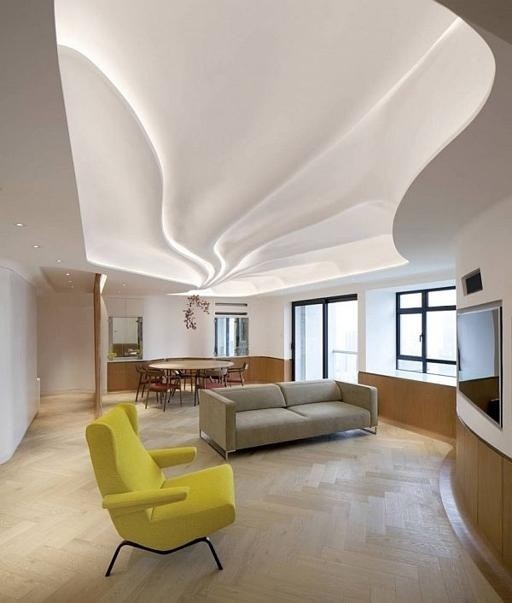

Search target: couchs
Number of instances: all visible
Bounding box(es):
[199,379,378,460]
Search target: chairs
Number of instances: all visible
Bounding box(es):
[86,403,236,577]
[135,359,249,412]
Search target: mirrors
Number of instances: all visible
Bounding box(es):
[110,317,143,359]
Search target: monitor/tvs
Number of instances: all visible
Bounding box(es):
[456,301,503,429]
